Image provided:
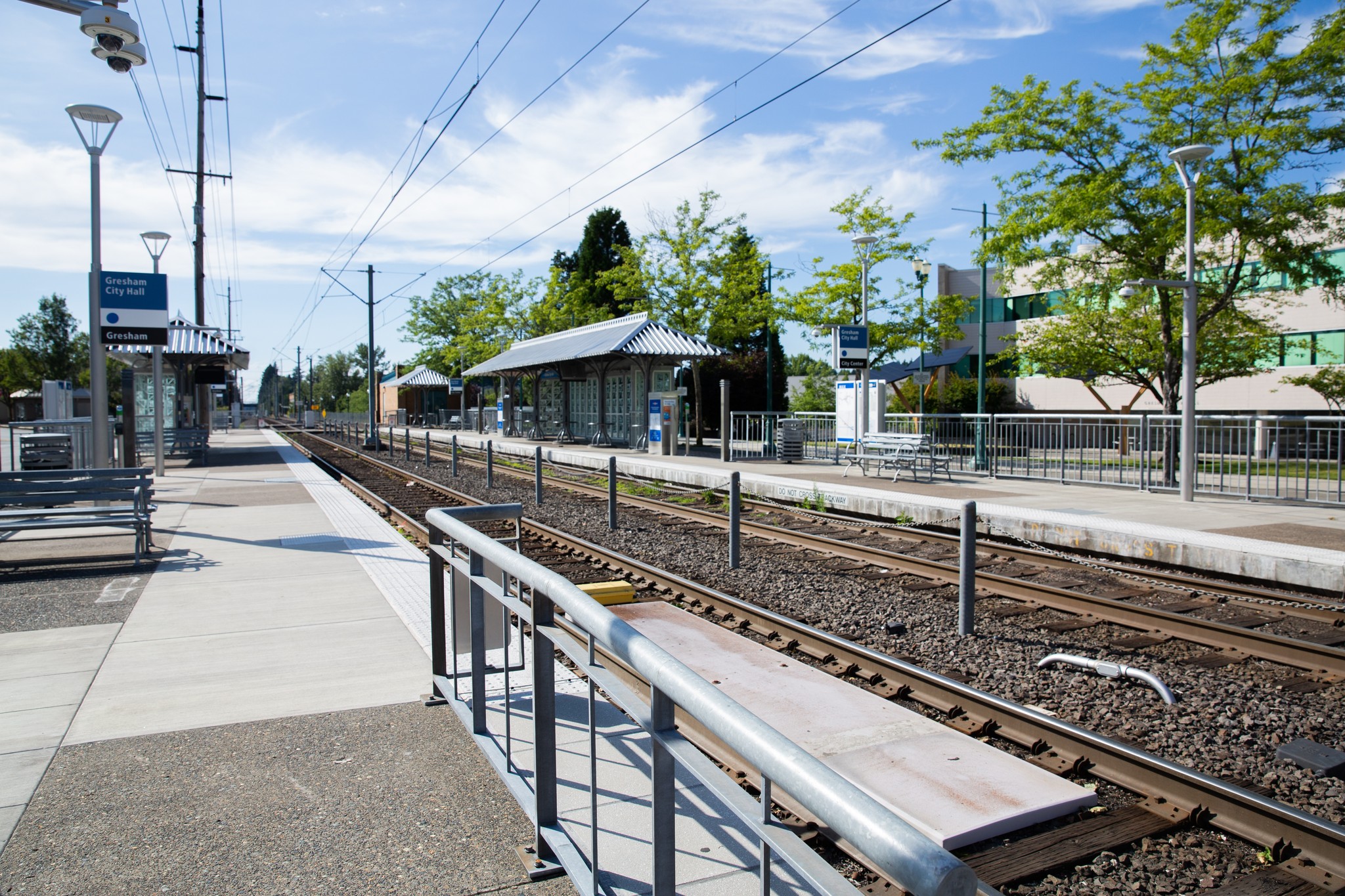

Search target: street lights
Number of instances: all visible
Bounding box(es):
[140,231,171,477]
[912,255,932,435]
[65,103,124,517]
[1167,144,1214,500]
[852,235,880,473]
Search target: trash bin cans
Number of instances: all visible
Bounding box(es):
[19,433,75,505]
[776,418,804,464]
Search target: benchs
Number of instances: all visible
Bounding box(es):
[458,416,472,432]
[0,468,158,552]
[134,430,208,467]
[876,433,953,481]
[840,432,924,483]
[0,466,151,568]
[441,416,459,432]
